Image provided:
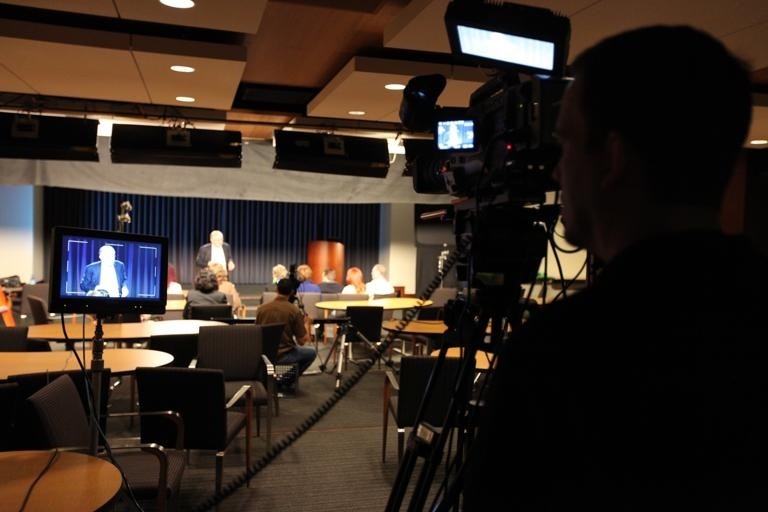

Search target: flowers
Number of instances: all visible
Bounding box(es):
[116,200,132,233]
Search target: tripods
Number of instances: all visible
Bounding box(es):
[319,323,401,392]
[288,295,323,366]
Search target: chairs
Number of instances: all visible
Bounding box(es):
[0,283,476,512]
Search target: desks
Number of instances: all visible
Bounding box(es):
[430,347,498,373]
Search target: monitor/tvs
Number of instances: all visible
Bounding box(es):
[46,225,169,316]
[444,1,571,77]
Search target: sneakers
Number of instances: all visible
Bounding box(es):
[275,380,296,399]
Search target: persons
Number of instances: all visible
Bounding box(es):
[253,278,317,397]
[341,266,373,295]
[185,267,228,320]
[195,229,235,271]
[271,263,290,284]
[79,245,129,298]
[296,264,321,294]
[205,263,242,313]
[457,24,768,511]
[366,263,395,295]
[319,268,342,294]
